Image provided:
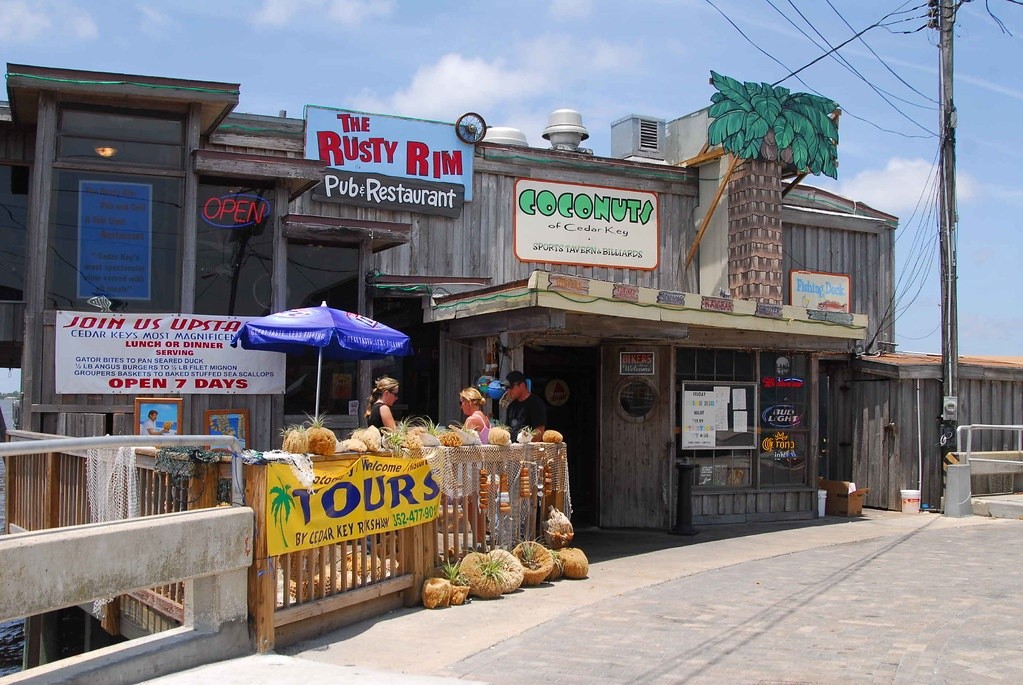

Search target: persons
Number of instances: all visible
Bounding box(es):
[365,377,399,553]
[459,370,547,555]
[142,410,169,435]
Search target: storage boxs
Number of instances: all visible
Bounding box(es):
[820,480,872,517]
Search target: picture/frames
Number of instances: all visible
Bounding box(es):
[134,398,184,435]
[203,408,250,453]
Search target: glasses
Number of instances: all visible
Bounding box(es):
[384,389,398,398]
[505,383,520,390]
[458,399,467,407]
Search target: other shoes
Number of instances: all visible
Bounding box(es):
[485,534,497,545]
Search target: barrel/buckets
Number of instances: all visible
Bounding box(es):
[900,489,921,515]
[817,489,827,517]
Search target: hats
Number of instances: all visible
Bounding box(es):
[500,370,525,387]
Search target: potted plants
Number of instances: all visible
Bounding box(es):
[276,420,309,454]
[438,533,563,605]
[379,413,540,459]
[300,410,336,456]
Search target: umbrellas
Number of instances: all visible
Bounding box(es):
[230,301,414,418]
